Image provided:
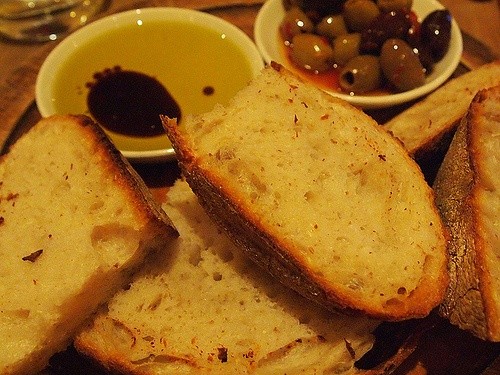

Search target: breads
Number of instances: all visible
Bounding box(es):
[0,61,500,375]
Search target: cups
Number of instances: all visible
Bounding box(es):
[0,0,103,42]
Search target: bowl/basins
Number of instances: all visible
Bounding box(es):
[34,6,265,161]
[253,0,463,107]
